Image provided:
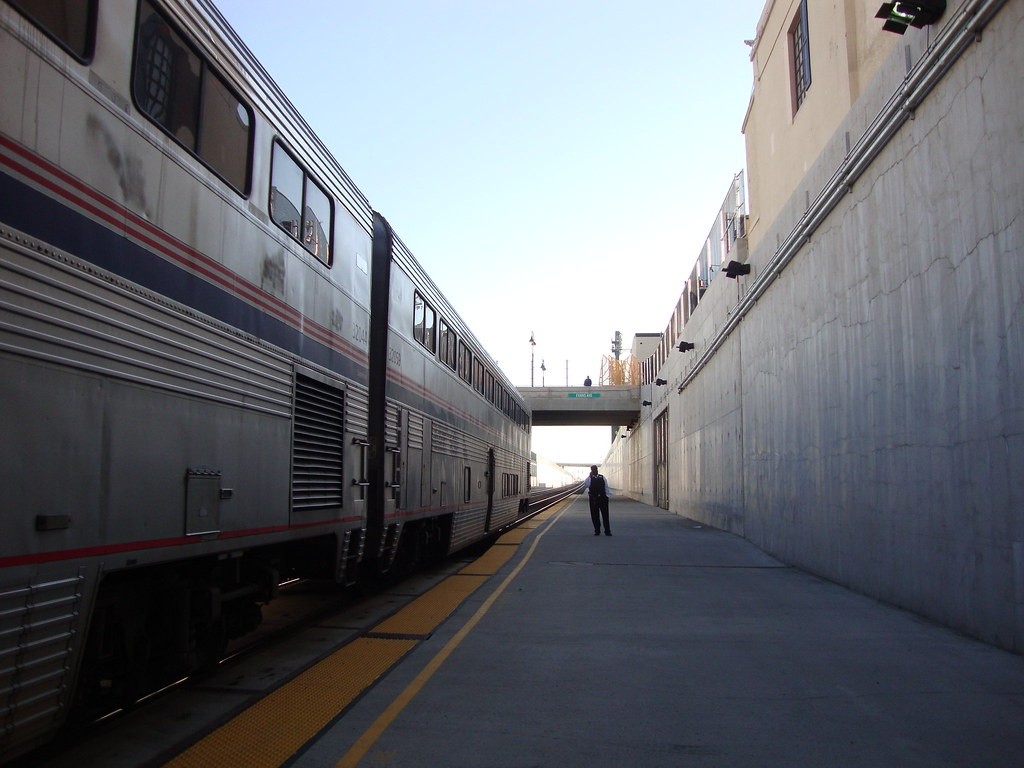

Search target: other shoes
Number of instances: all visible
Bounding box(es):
[595,531,600,536]
[606,533,612,537]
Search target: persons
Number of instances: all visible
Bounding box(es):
[584,376,592,386]
[584,465,612,536]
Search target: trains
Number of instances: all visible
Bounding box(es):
[0,0,532,768]
